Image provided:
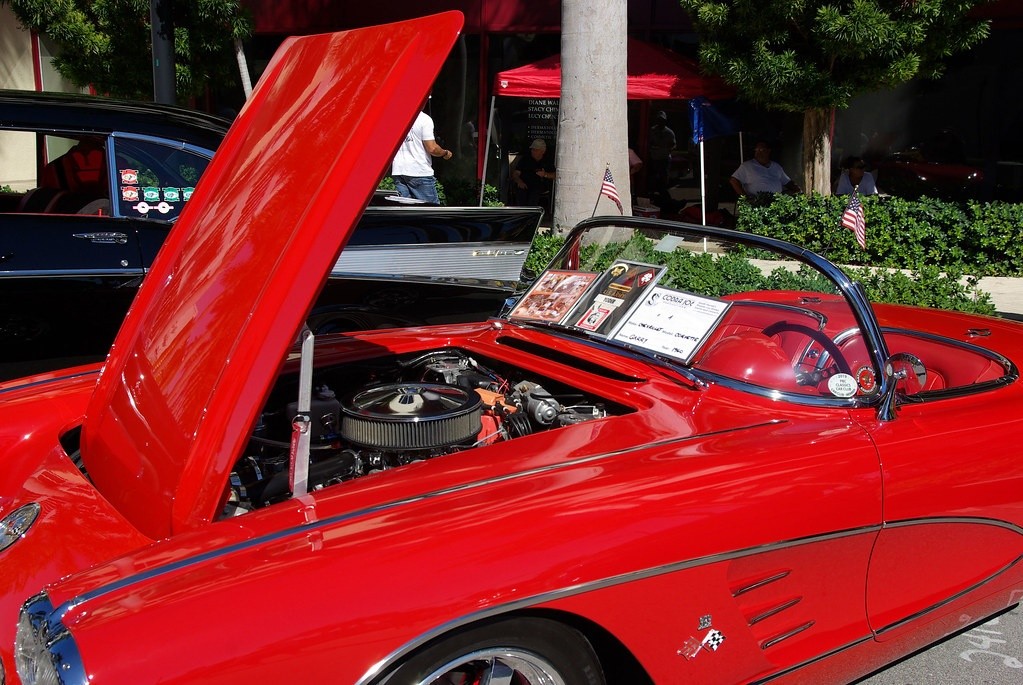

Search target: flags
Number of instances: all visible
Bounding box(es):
[839,191,867,253]
[598,167,624,215]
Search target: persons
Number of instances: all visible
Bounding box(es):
[730,141,804,208]
[223,108,236,121]
[646,109,677,203]
[835,130,882,196]
[512,138,556,216]
[629,147,642,182]
[391,88,452,204]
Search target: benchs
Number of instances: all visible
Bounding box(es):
[18,186,109,215]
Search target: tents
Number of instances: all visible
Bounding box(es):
[479,35,739,226]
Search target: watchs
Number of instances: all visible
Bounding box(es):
[544,172,548,178]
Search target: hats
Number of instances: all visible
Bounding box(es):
[656,110,667,121]
[530,139,547,149]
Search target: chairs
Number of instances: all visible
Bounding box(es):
[818,333,1004,398]
[692,305,820,378]
[505,152,524,205]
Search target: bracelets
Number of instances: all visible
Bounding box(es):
[441,150,448,157]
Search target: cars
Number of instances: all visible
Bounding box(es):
[0,11,1023,685]
[0,89,439,382]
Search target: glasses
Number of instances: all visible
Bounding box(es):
[849,165,867,171]
[753,147,768,152]
